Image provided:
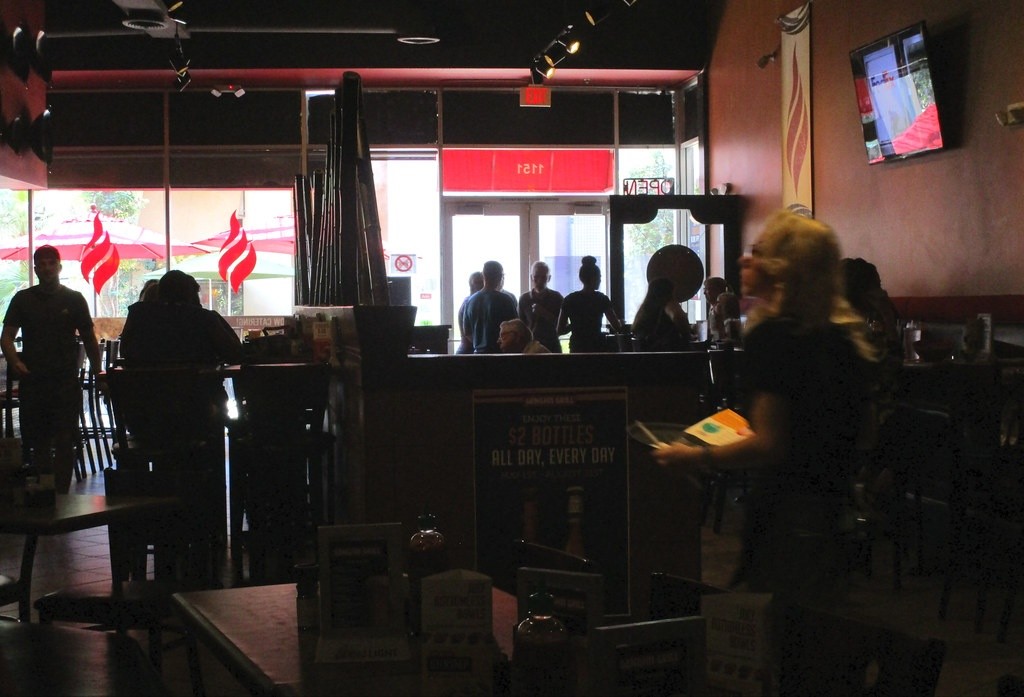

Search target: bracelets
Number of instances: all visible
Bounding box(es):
[702,447,718,468]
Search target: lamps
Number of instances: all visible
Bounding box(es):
[169,19,193,91]
[530,0,637,85]
[755,53,775,70]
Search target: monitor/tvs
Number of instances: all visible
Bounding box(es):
[849,19,949,165]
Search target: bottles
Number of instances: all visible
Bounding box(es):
[409,517,447,625]
[511,592,572,697]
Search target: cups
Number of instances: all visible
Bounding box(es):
[696,321,708,341]
[904,328,921,361]
[248,329,260,339]
[232,327,243,344]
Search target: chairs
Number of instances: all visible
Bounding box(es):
[0,322,1024,697]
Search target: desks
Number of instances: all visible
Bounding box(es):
[170,573,517,697]
[0,623,177,697]
[0,496,180,670]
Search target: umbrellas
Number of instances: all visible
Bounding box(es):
[144,248,294,316]
[0,202,390,316]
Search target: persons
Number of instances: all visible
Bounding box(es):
[463,260,518,354]
[122,269,241,450]
[651,210,865,697]
[0,245,110,493]
[705,256,754,345]
[497,319,551,353]
[633,276,690,352]
[557,256,622,353]
[520,260,566,353]
[457,273,484,353]
[831,257,957,501]
